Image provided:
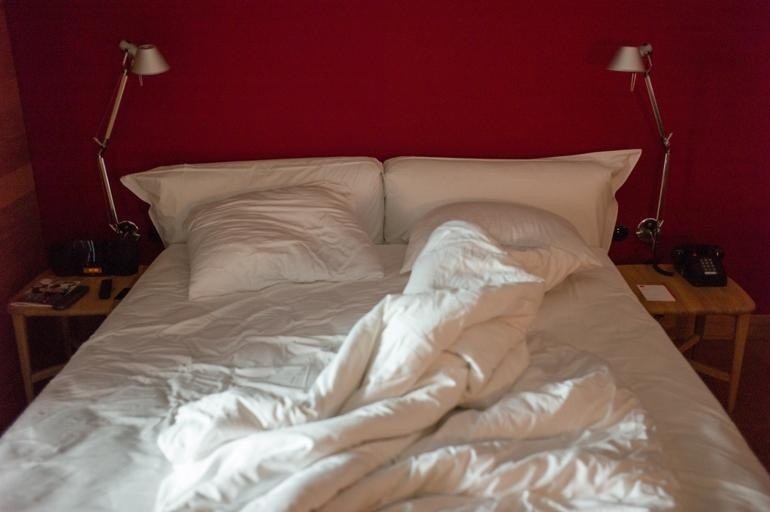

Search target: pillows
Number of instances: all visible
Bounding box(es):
[381,149,644,248]
[399,200,606,279]
[119,155,385,248]
[182,178,389,301]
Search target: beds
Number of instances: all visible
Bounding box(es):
[0,246,768,510]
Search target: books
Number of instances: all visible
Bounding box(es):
[10,276,81,308]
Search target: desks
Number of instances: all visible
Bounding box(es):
[616,261,756,413]
[7,266,145,407]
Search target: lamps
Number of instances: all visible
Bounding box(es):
[92,41,172,240]
[607,44,677,243]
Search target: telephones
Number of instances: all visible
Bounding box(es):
[671,244,727,287]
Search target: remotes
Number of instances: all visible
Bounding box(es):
[99,279,112,299]
[53,285,89,310]
